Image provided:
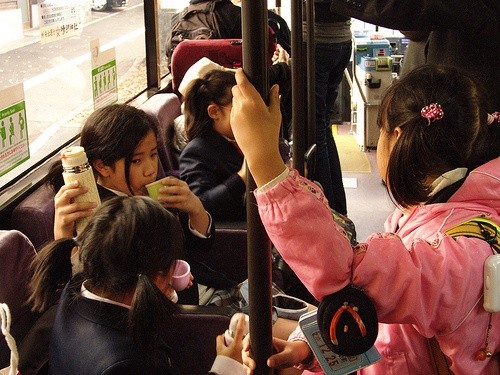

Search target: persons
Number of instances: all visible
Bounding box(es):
[230,64,500,375]
[330,0,500,100]
[26,0,318,375]
[301,0,352,216]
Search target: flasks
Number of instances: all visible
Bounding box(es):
[59,146,102,236]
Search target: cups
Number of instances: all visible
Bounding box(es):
[167,259,190,291]
[145,176,177,204]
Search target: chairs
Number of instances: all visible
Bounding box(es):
[0,39,247,375]
